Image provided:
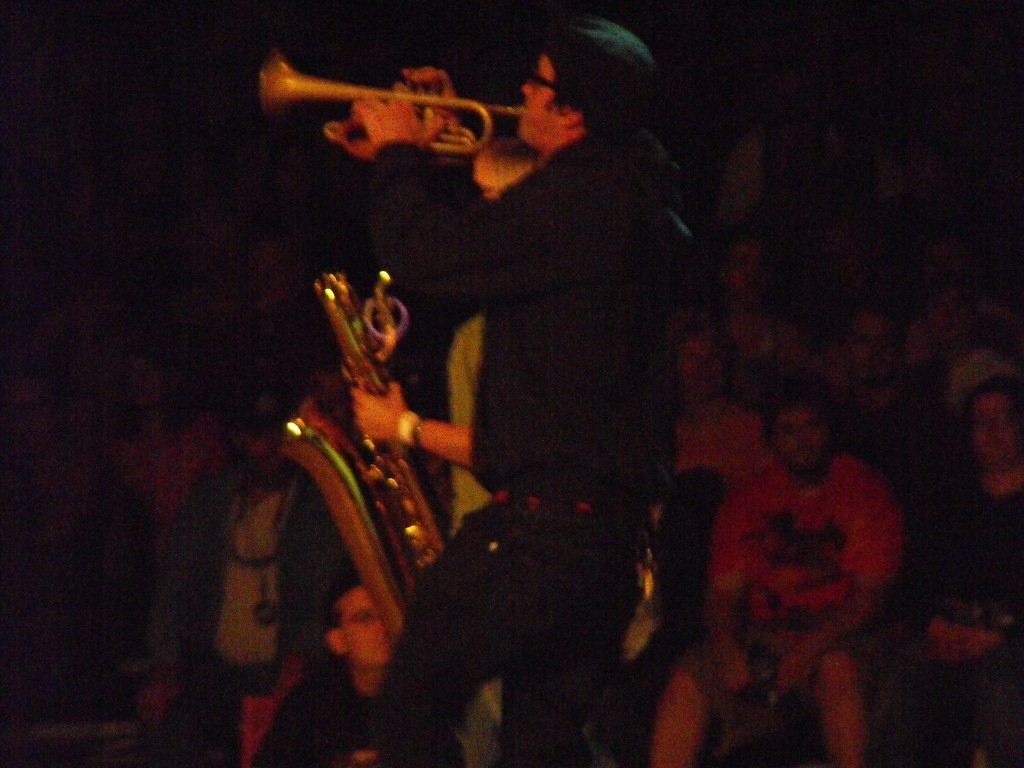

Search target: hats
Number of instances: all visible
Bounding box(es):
[560,13,660,128]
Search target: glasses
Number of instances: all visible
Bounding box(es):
[526,66,558,91]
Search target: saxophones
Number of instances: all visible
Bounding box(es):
[315,264,449,569]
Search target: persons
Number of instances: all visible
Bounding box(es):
[100,233,1024,768]
[347,17,693,768]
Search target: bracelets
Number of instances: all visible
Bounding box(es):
[395,411,419,445]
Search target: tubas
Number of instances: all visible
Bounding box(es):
[278,399,660,731]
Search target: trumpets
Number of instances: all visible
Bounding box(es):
[258,45,525,165]
